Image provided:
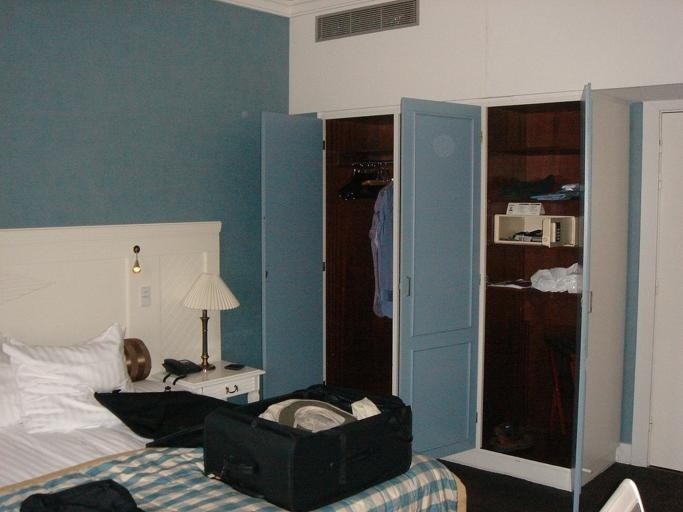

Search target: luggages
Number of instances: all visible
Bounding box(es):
[94,384,412,512]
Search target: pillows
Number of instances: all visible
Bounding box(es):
[123,336,151,382]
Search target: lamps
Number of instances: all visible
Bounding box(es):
[182,273,240,371]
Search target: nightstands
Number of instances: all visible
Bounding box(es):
[151,360,267,404]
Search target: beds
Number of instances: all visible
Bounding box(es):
[0,221,467,512]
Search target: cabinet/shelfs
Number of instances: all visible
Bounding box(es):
[262,82,630,512]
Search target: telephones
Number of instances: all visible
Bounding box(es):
[162,359,203,376]
